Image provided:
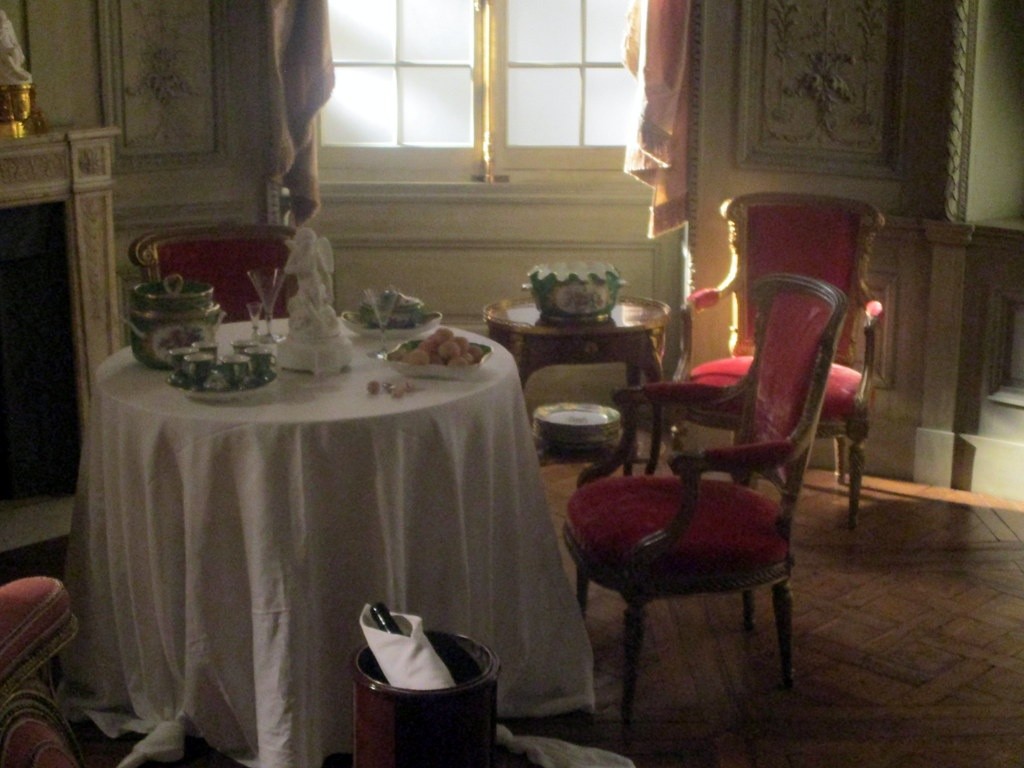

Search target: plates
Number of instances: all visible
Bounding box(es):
[383,339,493,379]
[341,310,442,339]
[164,376,279,399]
[532,402,623,451]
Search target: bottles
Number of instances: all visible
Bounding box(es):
[370,601,405,636]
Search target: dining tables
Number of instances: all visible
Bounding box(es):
[63,315,599,768]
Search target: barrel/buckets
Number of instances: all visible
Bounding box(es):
[355,630,500,768]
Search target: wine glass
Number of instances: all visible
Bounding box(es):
[364,287,398,358]
[247,269,286,341]
[247,302,264,339]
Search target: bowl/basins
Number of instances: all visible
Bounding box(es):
[521,260,628,324]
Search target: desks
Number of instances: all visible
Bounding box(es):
[482,294,679,489]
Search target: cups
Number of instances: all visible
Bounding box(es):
[219,355,251,385]
[230,340,259,355]
[244,347,277,375]
[168,349,193,379]
[185,353,215,387]
[192,341,218,368]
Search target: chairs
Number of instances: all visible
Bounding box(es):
[650,190,885,531]
[560,272,847,729]
[127,220,297,322]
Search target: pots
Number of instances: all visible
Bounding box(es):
[120,281,227,371]
